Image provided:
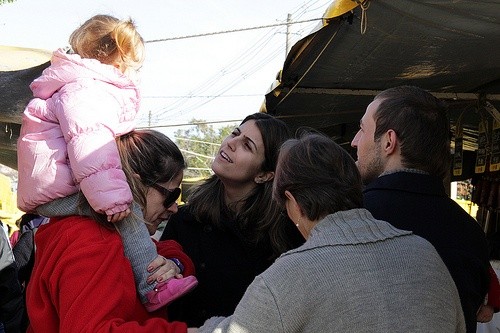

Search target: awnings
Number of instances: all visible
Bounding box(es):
[255,0,500,180]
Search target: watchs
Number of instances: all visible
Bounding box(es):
[170,258,185,277]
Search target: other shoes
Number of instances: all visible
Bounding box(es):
[141,275,197,312]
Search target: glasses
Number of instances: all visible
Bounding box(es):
[143,178,181,208]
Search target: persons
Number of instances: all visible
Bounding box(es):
[17,15,199,313]
[159,112,307,328]
[198,126,467,332]
[477,303,494,323]
[26,130,202,333]
[0,215,34,333]
[351,87,491,333]
